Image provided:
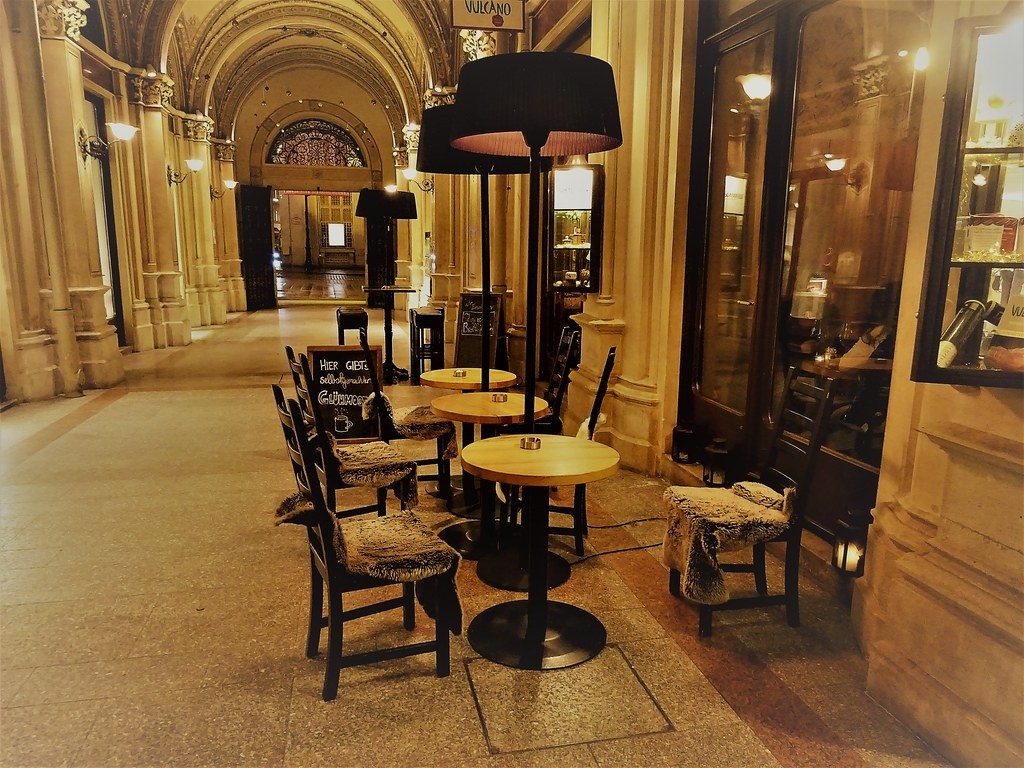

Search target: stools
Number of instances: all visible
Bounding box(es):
[336,306,369,347]
[406,306,448,384]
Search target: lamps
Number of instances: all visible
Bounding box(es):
[733,36,774,98]
[449,52,625,596]
[84,123,140,157]
[226,135,233,144]
[167,160,204,185]
[357,188,418,379]
[404,168,435,193]
[722,173,747,216]
[383,182,399,193]
[210,180,239,201]
[458,28,497,61]
[401,119,422,154]
[564,155,593,242]
[147,64,157,78]
[820,153,868,190]
[196,108,204,119]
[830,524,868,584]
[414,97,551,558]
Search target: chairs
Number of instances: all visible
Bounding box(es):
[504,324,580,436]
[271,381,461,708]
[499,346,617,550]
[285,345,417,515]
[660,368,837,638]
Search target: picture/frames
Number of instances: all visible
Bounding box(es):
[327,223,346,247]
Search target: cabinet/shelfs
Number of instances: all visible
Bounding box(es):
[939,147,1023,370]
[818,283,890,347]
[549,163,605,293]
[717,169,750,293]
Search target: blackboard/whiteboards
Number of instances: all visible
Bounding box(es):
[305,344,384,444]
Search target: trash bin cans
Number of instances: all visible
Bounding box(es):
[453,286,502,369]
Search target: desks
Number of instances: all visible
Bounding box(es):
[360,286,417,386]
[420,368,518,497]
[318,246,357,267]
[827,357,896,445]
[459,434,621,669]
[430,391,548,562]
